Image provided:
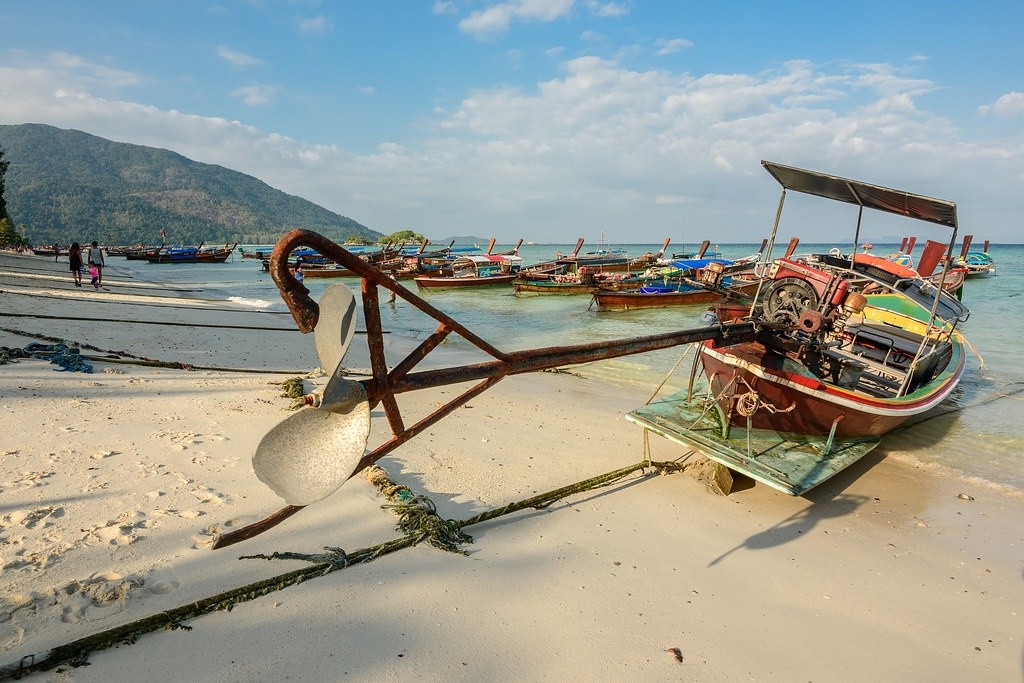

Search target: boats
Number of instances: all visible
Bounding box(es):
[697,161,970,443]
[28,235,971,328]
[947,235,996,279]
